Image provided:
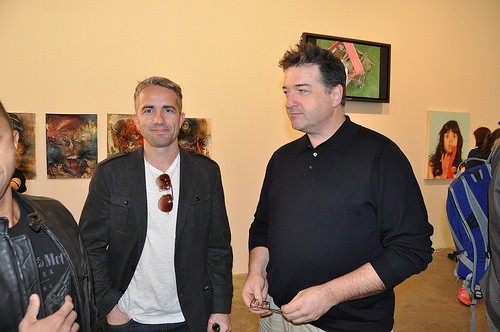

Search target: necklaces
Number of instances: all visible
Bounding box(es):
[144,156,179,185]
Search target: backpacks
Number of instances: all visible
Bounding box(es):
[446,138,500,300]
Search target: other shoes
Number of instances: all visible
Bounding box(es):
[457,288,472,305]
[448,251,460,262]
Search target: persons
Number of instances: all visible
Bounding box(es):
[427,120,465,179]
[448,122,500,332]
[79,77,233,332]
[0,102,100,332]
[241,41,434,332]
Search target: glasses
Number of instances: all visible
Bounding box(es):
[250,278,283,314]
[156,173,173,214]
